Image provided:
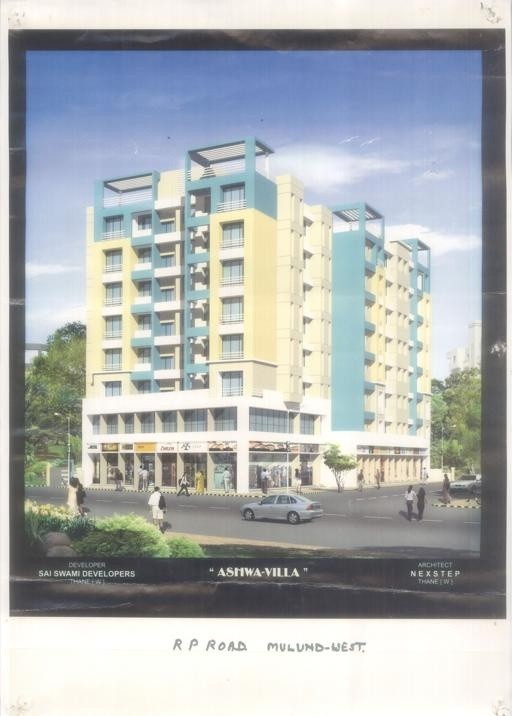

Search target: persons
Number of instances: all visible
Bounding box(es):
[295,469,302,493]
[374,469,380,489]
[405,485,429,521]
[442,474,452,504]
[196,471,204,493]
[177,472,190,496]
[148,487,167,530]
[357,469,364,490]
[61,477,86,517]
[222,468,230,493]
[138,466,149,490]
[115,470,123,491]
[260,469,269,493]
